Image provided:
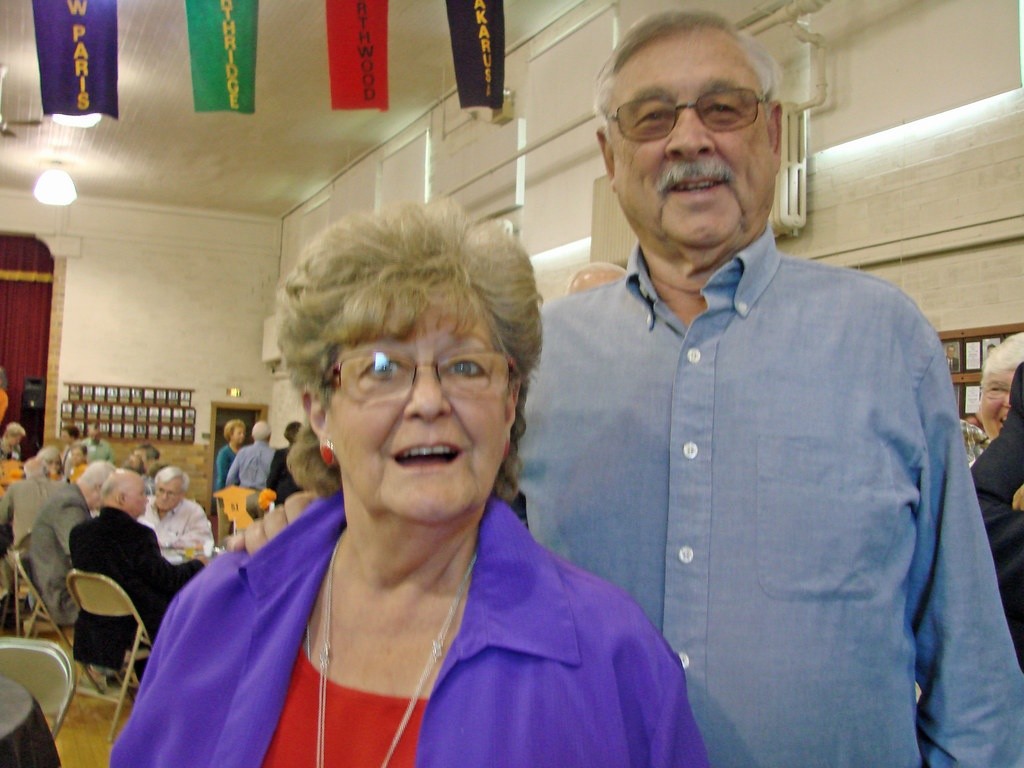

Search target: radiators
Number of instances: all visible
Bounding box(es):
[262,311,287,377]
[592,101,809,267]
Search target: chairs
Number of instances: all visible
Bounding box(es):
[0,530,153,742]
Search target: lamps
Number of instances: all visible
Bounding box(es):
[32,161,77,205]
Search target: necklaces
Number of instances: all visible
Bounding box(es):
[317,530,477,768]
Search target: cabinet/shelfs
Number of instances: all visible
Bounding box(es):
[936,322,1024,429]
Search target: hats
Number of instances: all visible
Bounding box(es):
[252,423,271,442]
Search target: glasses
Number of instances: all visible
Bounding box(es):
[319,348,517,402]
[604,88,765,141]
[980,382,1013,399]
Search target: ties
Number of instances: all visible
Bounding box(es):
[949,359,951,369]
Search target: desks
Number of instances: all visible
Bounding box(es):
[0,675,62,768]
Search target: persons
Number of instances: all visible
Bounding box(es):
[0,422,159,612]
[108,203,712,768]
[138,466,215,550]
[68,468,207,692]
[227,9,1024,768]
[216,420,304,546]
[971,363,1024,673]
[31,459,117,626]
[987,344,995,356]
[946,344,959,372]
[961,333,1024,466]
